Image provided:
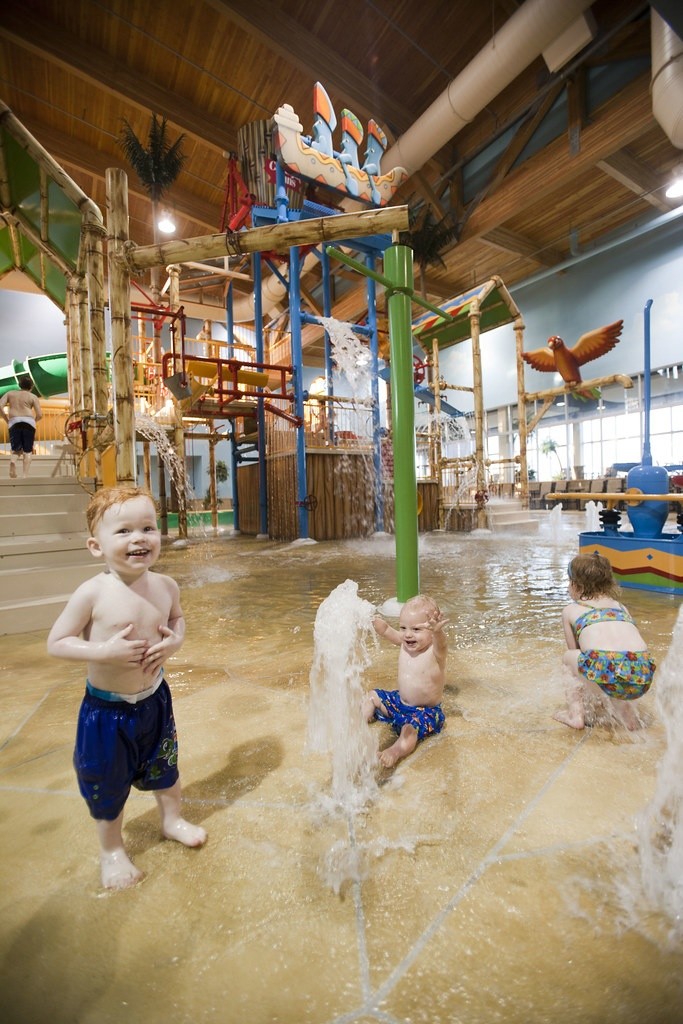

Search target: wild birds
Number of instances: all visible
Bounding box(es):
[520,319,624,400]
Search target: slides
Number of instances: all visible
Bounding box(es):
[0,352,146,400]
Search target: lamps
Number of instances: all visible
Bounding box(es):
[666,162,683,198]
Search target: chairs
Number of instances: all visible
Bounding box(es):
[528,477,625,511]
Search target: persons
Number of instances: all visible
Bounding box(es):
[550,553,656,730]
[0,376,42,478]
[46,485,207,888]
[361,594,450,768]
